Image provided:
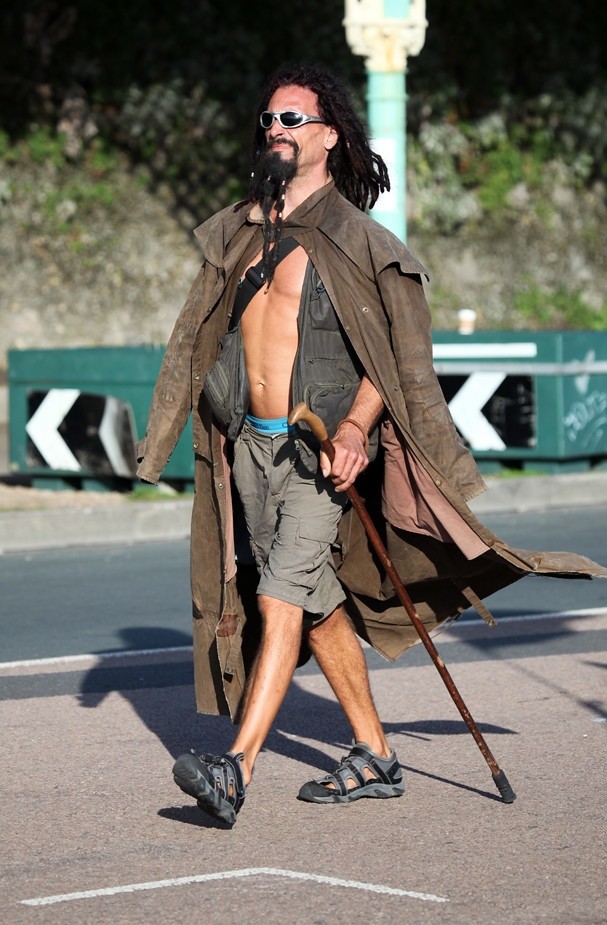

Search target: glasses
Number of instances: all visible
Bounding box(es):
[259,111,324,129]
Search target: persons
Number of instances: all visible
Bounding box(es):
[171,67,431,826]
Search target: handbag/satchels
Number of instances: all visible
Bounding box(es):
[202,236,300,441]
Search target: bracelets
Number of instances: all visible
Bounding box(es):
[337,419,369,454]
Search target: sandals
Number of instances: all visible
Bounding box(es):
[299,737,405,804]
[172,748,248,825]
